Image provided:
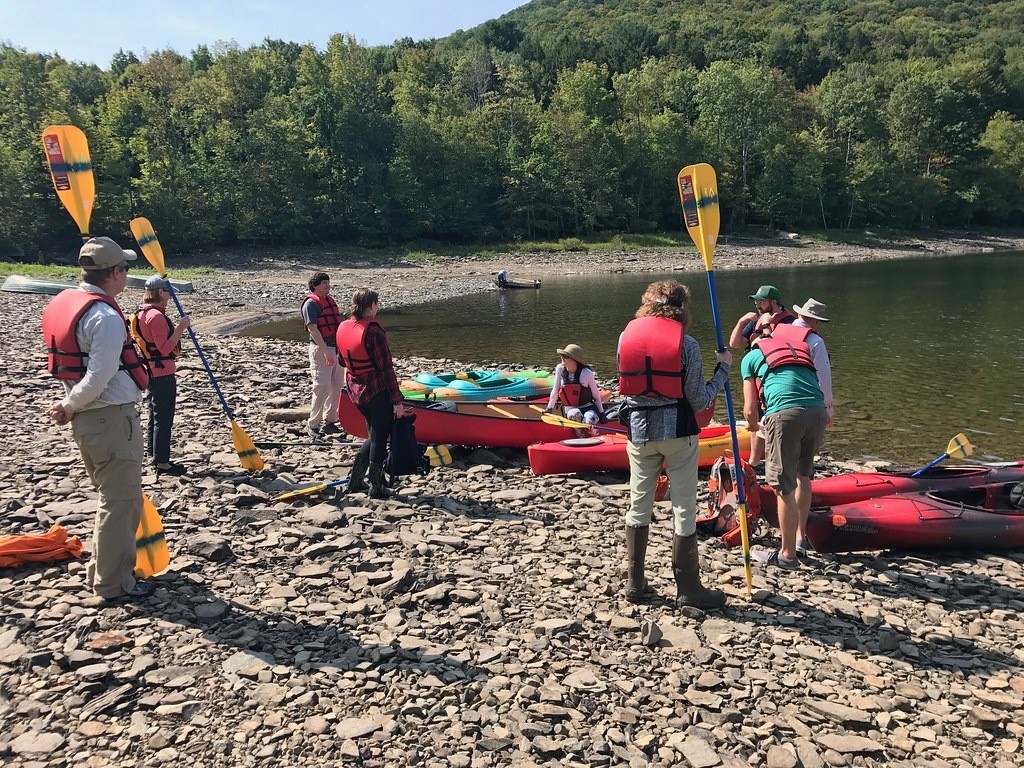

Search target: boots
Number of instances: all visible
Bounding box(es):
[370,460,396,499]
[672,531,727,609]
[625,524,657,601]
[347,453,372,493]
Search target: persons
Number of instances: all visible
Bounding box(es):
[130,275,190,475]
[548,344,607,439]
[42,237,156,599]
[617,281,733,610]
[335,288,405,497]
[729,286,834,570]
[301,272,344,438]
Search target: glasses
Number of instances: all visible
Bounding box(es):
[113,264,129,272]
[560,355,569,359]
[755,301,766,304]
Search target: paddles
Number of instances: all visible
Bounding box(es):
[41,125,170,578]
[270,444,453,500]
[677,161,754,598]
[129,217,265,472]
[540,415,628,434]
[911,433,973,477]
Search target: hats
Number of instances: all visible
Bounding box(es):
[793,298,831,323]
[557,344,587,366]
[78,237,137,270]
[748,286,781,304]
[144,275,179,293]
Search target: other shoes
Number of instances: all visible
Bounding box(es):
[152,461,187,476]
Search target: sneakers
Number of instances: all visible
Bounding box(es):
[325,424,342,433]
[108,578,156,601]
[308,428,325,438]
[752,550,798,570]
[795,538,807,557]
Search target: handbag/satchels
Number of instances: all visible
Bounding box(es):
[384,412,423,476]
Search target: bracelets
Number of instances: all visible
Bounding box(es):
[762,324,770,329]
